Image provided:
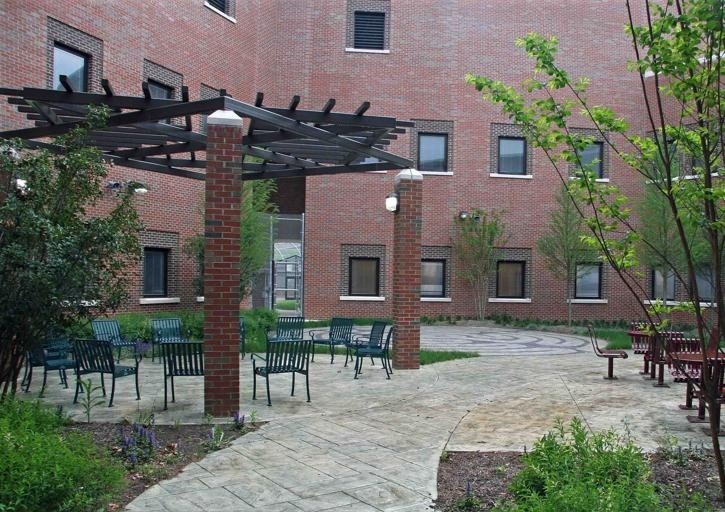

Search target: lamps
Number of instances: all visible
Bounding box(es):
[385,190,400,213]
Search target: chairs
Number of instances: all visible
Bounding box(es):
[584,320,628,382]
[627,323,724,440]
